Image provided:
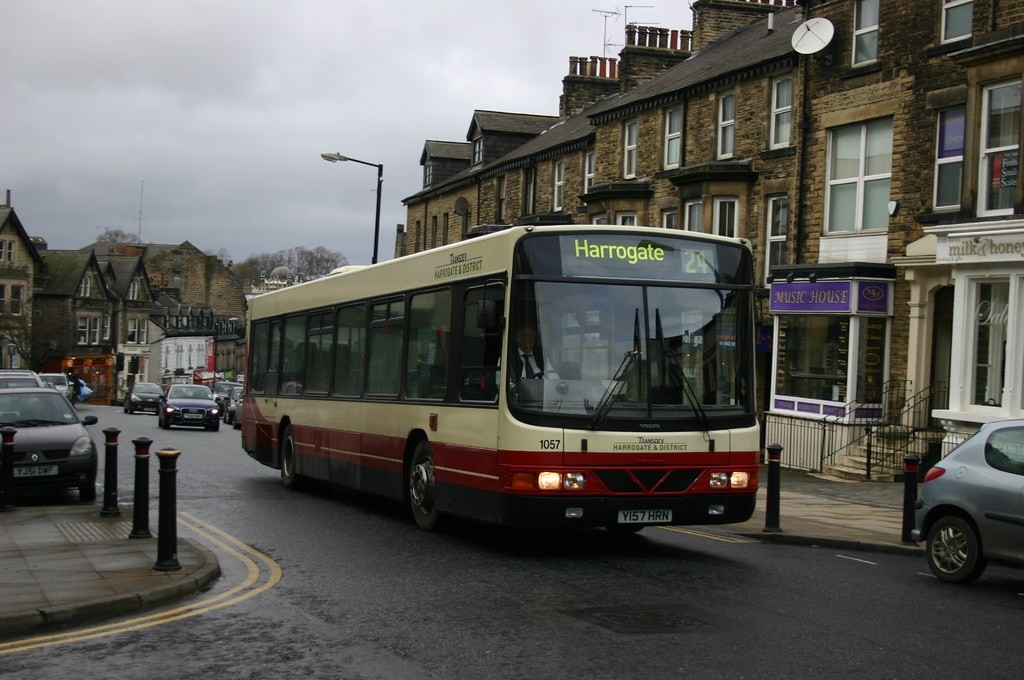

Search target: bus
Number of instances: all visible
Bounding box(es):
[239,222,761,533]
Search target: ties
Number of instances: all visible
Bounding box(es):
[522,354,535,379]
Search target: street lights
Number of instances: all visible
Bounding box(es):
[322,151,384,263]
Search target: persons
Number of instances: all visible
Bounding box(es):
[65,367,84,409]
[496,322,561,393]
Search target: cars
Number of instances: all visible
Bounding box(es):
[124,382,165,416]
[215,381,244,428]
[0,370,75,410]
[910,417,1024,586]
[0,388,100,505]
[158,384,223,432]
